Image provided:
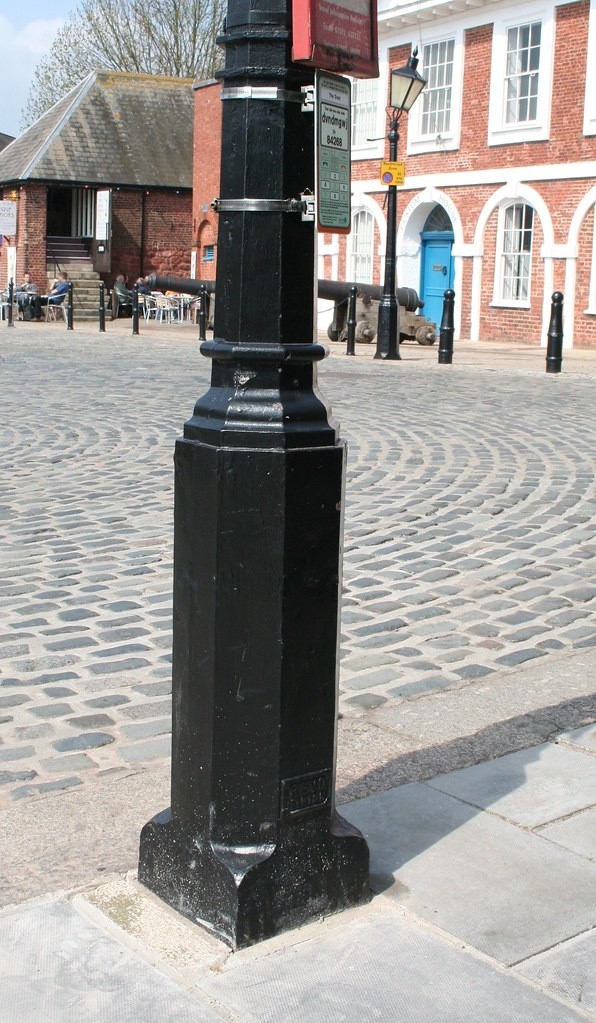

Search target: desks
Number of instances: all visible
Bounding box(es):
[170,297,194,323]
[14,292,37,321]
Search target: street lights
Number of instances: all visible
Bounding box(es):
[373,46,427,362]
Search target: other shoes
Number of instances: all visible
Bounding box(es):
[30,317,41,322]
[40,315,45,321]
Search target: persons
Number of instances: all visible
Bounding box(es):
[29,272,69,322]
[114,274,133,324]
[136,277,155,319]
[13,271,40,323]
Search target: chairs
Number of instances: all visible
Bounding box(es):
[41,292,67,322]
[109,290,133,317]
[138,293,201,323]
[0,290,11,321]
